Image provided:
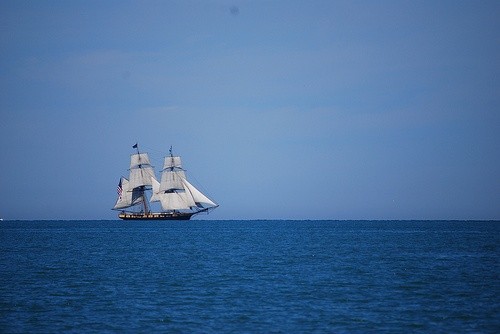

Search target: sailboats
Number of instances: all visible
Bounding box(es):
[111,143,220,220]
